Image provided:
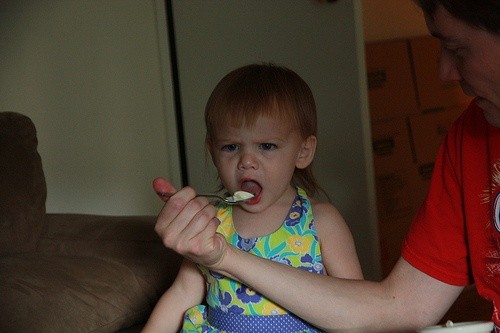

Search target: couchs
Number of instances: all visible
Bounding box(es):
[0,111,181,333]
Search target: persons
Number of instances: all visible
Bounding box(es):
[142,63,365,333]
[153,0,500,333]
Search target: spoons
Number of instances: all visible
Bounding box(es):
[158,191,255,204]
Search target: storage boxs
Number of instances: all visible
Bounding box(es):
[365,35,493,324]
